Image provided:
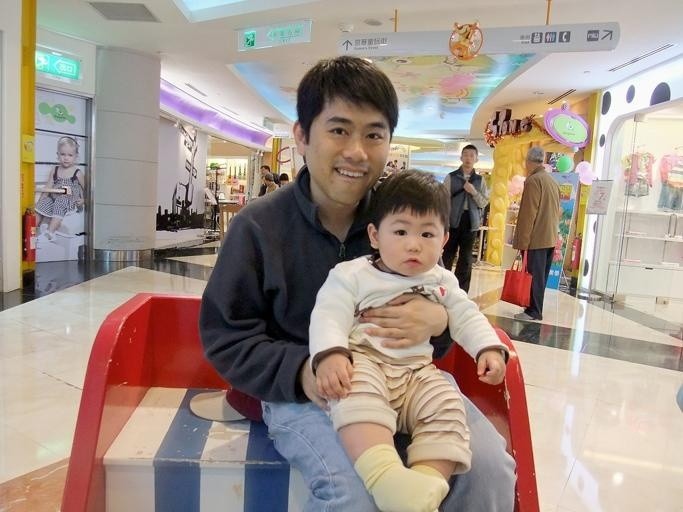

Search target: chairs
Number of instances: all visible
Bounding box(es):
[514,312,534,321]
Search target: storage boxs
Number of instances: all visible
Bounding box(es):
[58,289,540,512]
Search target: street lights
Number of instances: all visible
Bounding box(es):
[500,250,533,307]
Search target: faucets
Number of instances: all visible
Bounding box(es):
[37,230,55,242]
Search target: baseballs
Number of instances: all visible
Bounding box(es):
[570,233,582,269]
[22,208,37,262]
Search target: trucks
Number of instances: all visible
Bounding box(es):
[576,161,593,185]
[555,155,573,172]
[509,174,524,196]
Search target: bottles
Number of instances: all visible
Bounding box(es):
[266,172,274,181]
[279,173,289,180]
[190,384,263,423]
[527,147,545,162]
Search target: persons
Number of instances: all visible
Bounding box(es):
[383,161,393,177]
[440,145,490,295]
[305,167,511,512]
[276,173,289,186]
[391,160,397,168]
[196,54,522,512]
[510,145,563,321]
[257,165,281,197]
[262,172,279,195]
[400,161,406,169]
[33,136,85,243]
